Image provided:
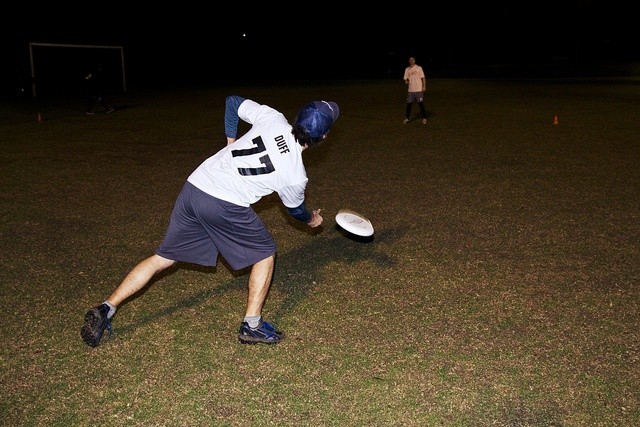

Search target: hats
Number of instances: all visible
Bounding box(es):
[297,100,340,138]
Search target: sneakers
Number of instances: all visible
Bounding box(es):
[422,119,427,124]
[403,119,409,123]
[238,318,282,345]
[80,304,113,349]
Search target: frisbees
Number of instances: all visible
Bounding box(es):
[335,212,374,236]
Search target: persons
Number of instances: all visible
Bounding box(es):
[80,95,341,348]
[402,56,429,125]
